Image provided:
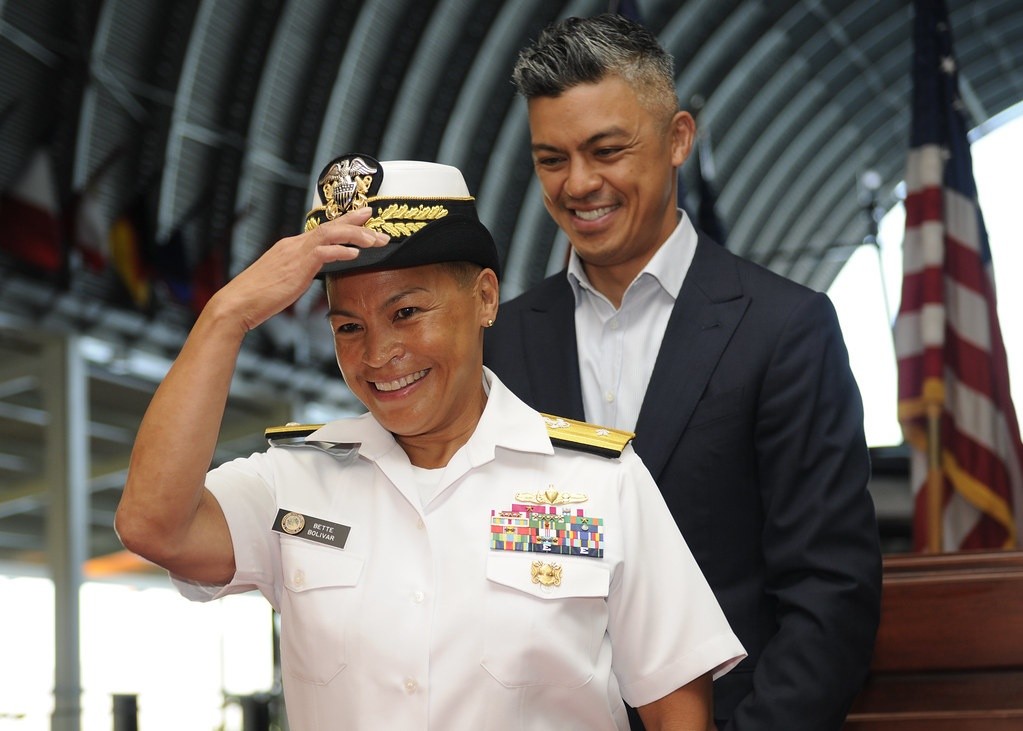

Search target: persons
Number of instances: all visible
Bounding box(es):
[481,19,887,731]
[113,153,748,731]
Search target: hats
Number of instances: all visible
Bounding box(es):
[300,153,499,280]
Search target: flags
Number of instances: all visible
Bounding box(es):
[890,0,1023,560]
